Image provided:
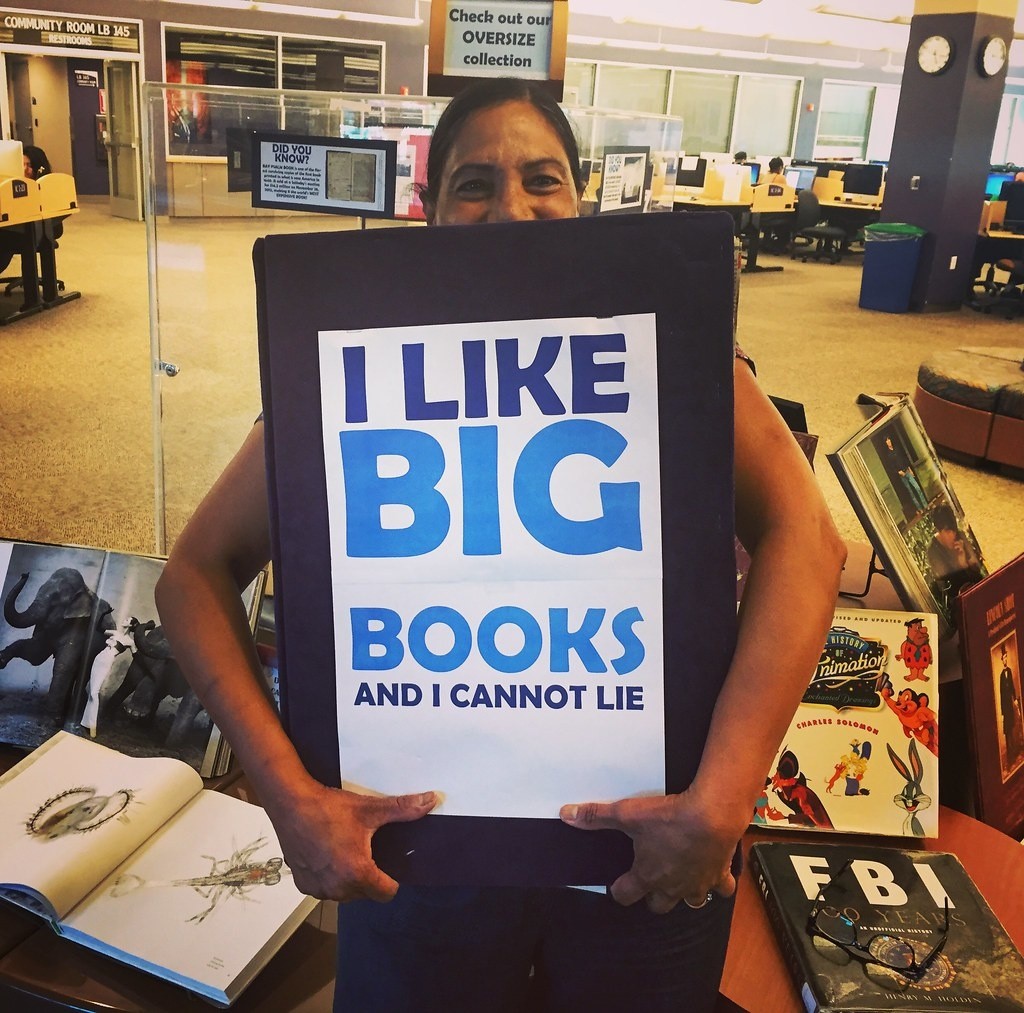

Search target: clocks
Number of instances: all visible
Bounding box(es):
[916,34,954,74]
[976,36,1007,79]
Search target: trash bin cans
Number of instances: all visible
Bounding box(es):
[857,221,929,315]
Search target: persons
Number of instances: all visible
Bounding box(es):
[0,147,59,270]
[152,79,850,1013]
[884,436,934,517]
[922,505,984,603]
[733,151,788,189]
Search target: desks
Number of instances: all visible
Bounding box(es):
[578,155,1024,311]
[0,173,80,327]
[0,771,1023,1013]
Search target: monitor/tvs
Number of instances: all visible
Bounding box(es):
[743,163,760,186]
[843,164,883,197]
[985,172,1015,199]
[782,165,818,192]
[676,158,707,188]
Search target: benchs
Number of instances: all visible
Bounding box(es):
[913,346,1024,481]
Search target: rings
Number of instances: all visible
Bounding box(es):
[683,891,712,909]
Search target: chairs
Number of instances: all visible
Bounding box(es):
[971,240,1024,318]
[797,191,847,265]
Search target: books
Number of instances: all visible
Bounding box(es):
[749,843,1024,1013]
[0,729,323,1006]
[748,392,1023,838]
[1,538,267,779]
